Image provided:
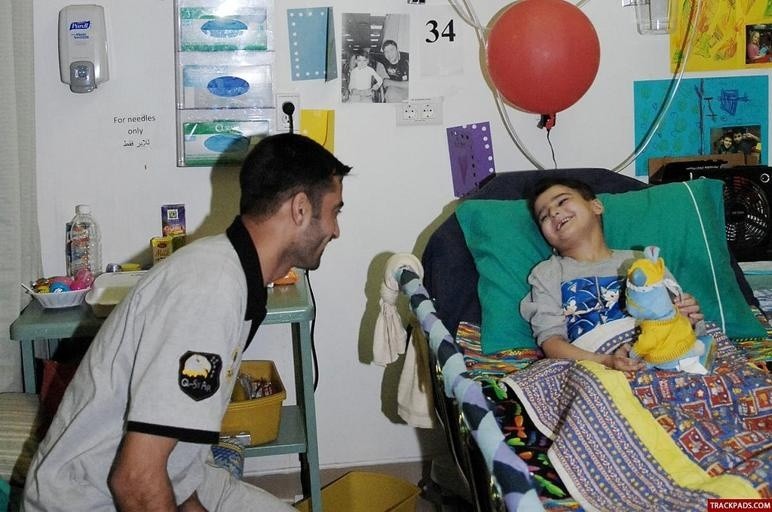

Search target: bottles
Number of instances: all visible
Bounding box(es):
[70,204,100,282]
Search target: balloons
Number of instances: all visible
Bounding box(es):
[485,1,601,132]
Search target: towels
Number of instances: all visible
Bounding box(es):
[371,250,439,430]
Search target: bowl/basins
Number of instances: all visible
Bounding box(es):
[25,286,92,308]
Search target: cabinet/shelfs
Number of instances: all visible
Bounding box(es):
[8,271,322,510]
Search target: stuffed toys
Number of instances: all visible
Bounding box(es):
[622,246,712,373]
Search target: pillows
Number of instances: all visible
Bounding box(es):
[454,177,769,357]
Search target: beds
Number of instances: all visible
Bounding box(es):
[395,164,770,512]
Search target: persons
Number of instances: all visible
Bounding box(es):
[18,131,355,512]
[517,175,704,374]
[344,31,409,103]
[720,128,760,154]
[718,134,737,154]
[748,29,771,63]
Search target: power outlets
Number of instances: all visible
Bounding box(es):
[275,95,300,133]
[394,97,445,127]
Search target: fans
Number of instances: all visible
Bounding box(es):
[687,166,771,264]
